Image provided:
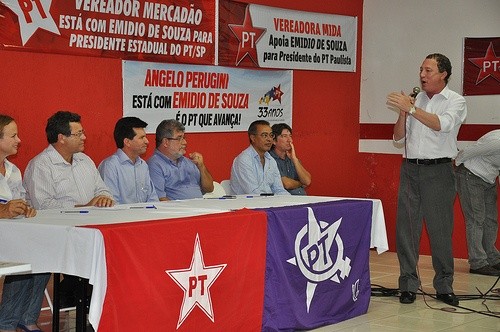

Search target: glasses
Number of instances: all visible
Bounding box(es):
[166,136,185,141]
[68,129,86,137]
[261,133,275,139]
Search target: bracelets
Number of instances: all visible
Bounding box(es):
[409,105,416,114]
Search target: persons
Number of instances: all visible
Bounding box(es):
[23,111,115,312]
[454,129,500,276]
[230,120,291,195]
[0,115,52,332]
[145,119,214,201]
[268,123,312,196]
[386,53,468,305]
[98,117,160,204]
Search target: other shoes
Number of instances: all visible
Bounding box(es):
[469,265,500,276]
[490,263,500,270]
[16,323,43,332]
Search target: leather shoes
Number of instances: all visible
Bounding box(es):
[437,292,459,306]
[400,292,416,303]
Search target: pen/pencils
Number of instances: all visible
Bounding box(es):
[60,210,89,214]
[204,197,236,199]
[153,205,158,209]
[129,206,154,209]
[223,195,253,198]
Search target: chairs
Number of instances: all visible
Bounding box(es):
[202,182,226,198]
[221,179,235,195]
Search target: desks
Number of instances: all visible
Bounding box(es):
[0,194,389,332]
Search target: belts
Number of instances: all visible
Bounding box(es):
[408,157,451,165]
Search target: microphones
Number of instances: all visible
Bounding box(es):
[411,87,420,98]
